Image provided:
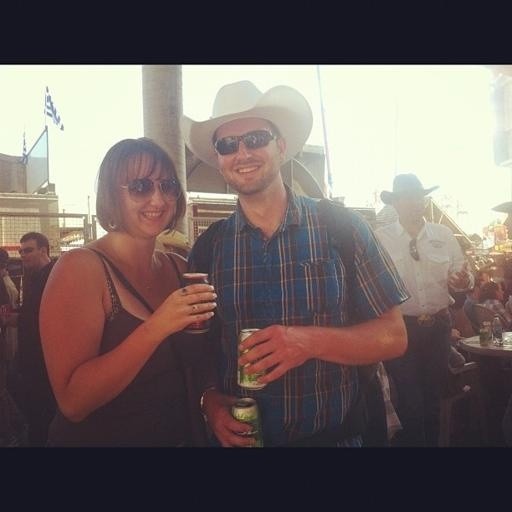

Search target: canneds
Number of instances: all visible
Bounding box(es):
[232,397,264,447]
[179,274,212,333]
[237,328,267,390]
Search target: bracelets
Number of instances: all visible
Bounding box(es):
[200,387,218,418]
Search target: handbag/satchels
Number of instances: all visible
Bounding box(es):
[358,362,407,443]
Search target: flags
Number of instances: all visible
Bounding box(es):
[45,90,65,132]
[22,135,30,165]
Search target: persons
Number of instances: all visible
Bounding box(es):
[0,249,21,361]
[18,233,54,340]
[39,138,217,446]
[373,174,474,446]
[178,78,411,447]
[480,282,511,325]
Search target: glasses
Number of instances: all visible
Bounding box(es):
[409,238,419,260]
[18,247,34,254]
[120,178,182,200]
[213,129,274,155]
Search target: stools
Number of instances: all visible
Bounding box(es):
[438,390,484,447]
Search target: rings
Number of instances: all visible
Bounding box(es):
[191,305,198,313]
[181,288,189,295]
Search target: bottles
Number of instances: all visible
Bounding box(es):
[182,272,214,334]
[491,313,503,346]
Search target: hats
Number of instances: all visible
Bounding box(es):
[381,175,438,204]
[181,80,313,169]
